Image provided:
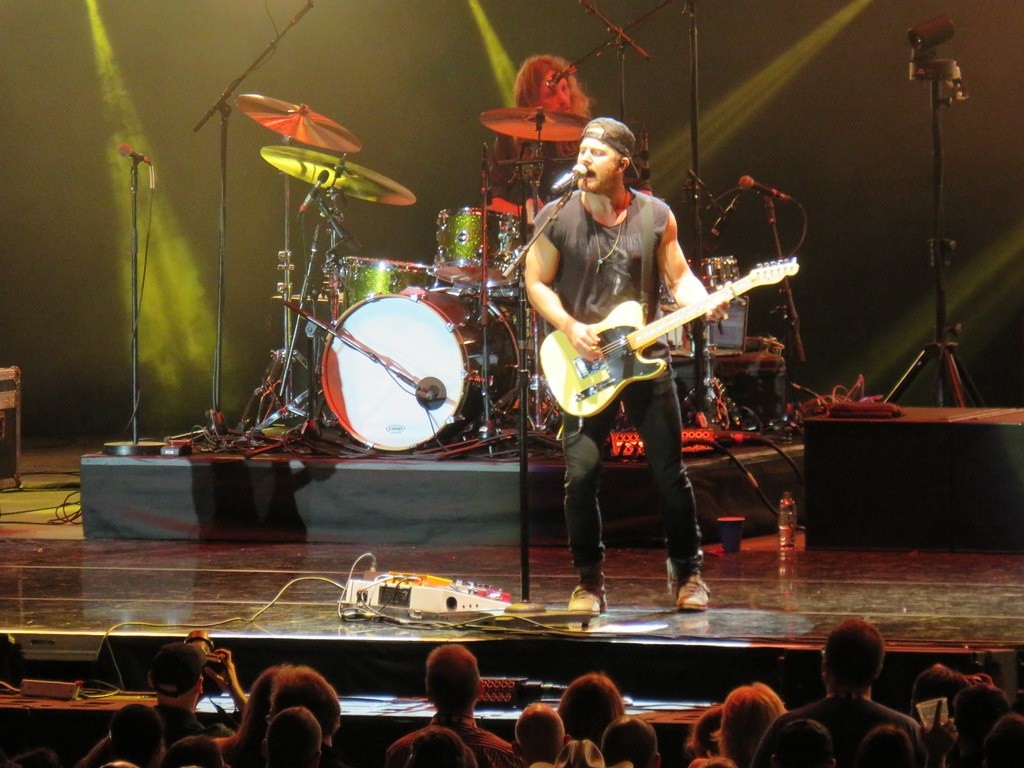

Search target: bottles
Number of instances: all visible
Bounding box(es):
[779,490,795,547]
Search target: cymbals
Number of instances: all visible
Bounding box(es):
[235,93,364,153]
[478,108,591,141]
[260,146,417,206]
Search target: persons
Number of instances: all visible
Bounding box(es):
[522,117,731,614]
[0,617,1024,768]
[480,53,666,225]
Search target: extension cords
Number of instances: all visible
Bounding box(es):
[20,680,80,700]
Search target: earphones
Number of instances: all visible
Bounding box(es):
[618,161,624,168]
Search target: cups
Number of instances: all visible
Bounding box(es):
[718,516,745,553]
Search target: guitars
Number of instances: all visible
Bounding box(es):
[538,255,801,419]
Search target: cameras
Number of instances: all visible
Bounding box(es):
[184,631,226,696]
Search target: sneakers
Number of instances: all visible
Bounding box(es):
[569,583,608,612]
[676,572,709,610]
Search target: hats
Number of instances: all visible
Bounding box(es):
[152,642,208,696]
[582,117,640,184]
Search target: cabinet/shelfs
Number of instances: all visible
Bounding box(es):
[0,368,26,491]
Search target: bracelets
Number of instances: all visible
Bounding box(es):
[516,203,521,219]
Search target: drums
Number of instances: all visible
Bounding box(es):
[321,288,520,452]
[432,206,536,289]
[337,256,454,328]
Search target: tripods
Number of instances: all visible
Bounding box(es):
[413,121,566,459]
[163,0,375,461]
[884,81,982,409]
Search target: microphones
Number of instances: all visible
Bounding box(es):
[907,16,957,51]
[704,196,740,254]
[640,128,651,180]
[739,175,793,202]
[545,72,568,91]
[117,143,150,166]
[551,163,588,196]
[426,384,438,401]
[482,143,494,206]
[298,170,329,214]
